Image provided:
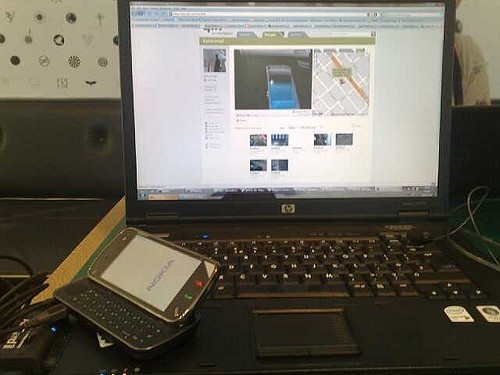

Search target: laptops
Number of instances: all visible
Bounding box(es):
[116,1,496,366]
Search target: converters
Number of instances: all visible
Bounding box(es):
[0,301,47,369]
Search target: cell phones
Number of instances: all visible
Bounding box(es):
[53,227,222,354]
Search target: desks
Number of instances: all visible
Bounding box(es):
[1,198,499,371]
